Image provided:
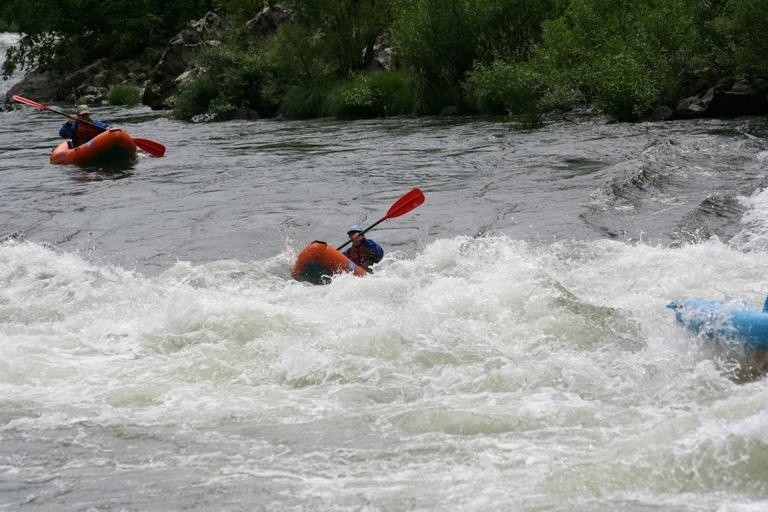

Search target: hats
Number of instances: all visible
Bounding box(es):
[346,225,364,234]
[77,105,90,114]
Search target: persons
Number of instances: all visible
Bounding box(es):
[58,102,112,146]
[340,224,384,269]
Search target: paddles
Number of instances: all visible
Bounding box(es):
[335,188,425,251]
[12,95,165,157]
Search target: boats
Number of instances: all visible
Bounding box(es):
[50,127,138,166]
[293,240,366,285]
[667,297,768,354]
